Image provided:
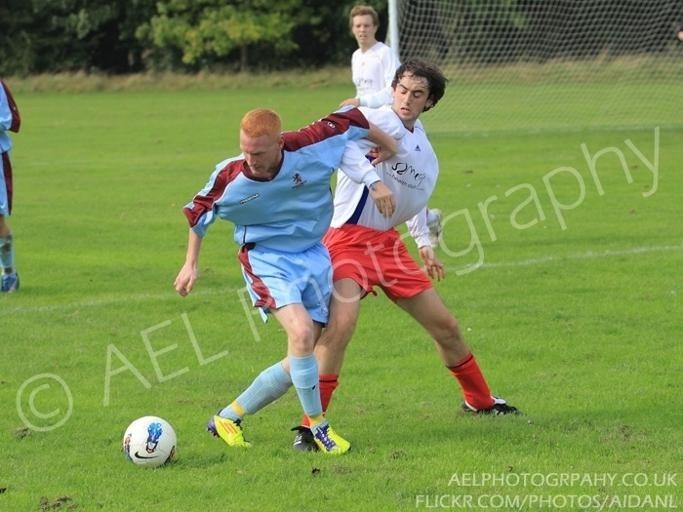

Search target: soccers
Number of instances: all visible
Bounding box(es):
[123,416,177,468]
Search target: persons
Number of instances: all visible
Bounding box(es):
[173,104,397,455]
[0,76,21,294]
[337,3,442,249]
[289,56,524,454]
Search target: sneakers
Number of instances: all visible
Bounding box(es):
[461,396,523,415]
[2,271,19,291]
[425,205,442,249]
[313,425,350,455]
[291,425,318,452]
[207,409,251,449]
